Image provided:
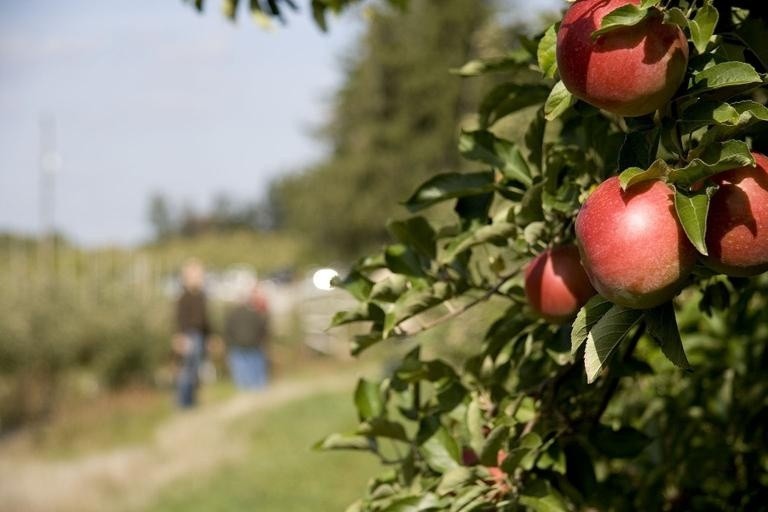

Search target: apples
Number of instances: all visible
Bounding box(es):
[575,175,698,309]
[698,152,767,278]
[527,244,594,324]
[557,1,689,117]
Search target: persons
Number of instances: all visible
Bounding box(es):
[222,297,268,391]
[171,257,211,409]
